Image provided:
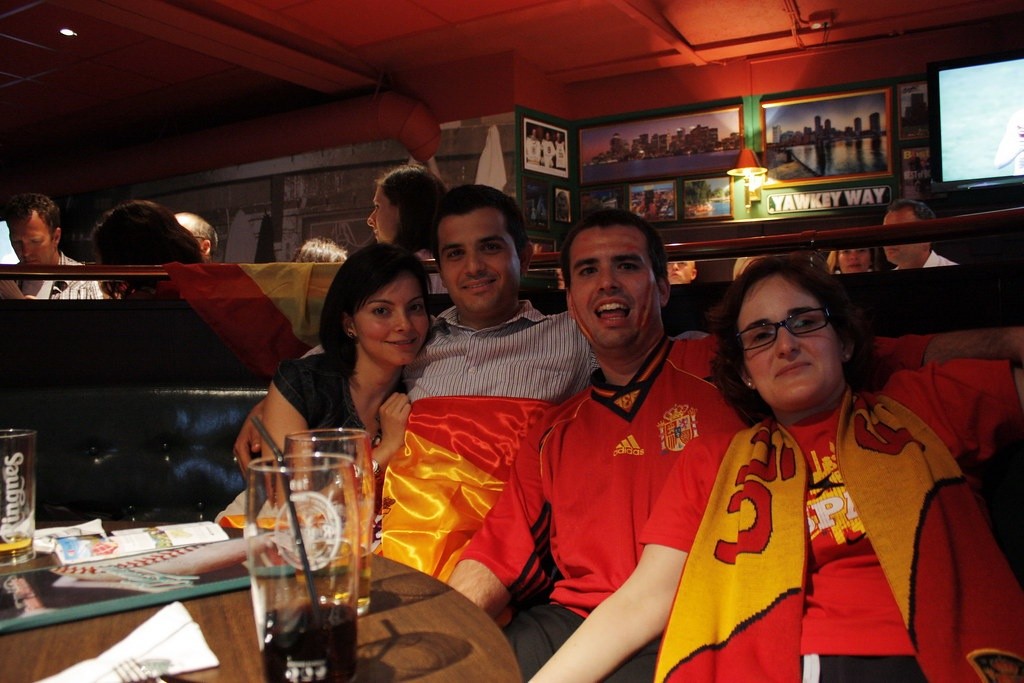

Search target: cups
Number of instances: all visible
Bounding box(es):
[244,452,358,683]
[0,429,37,568]
[281,429,375,616]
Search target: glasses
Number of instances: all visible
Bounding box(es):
[737,307,830,350]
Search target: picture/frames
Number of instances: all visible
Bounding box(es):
[578,187,626,220]
[555,186,574,224]
[897,146,936,201]
[578,103,747,187]
[681,175,730,221]
[628,180,679,223]
[520,112,571,180]
[896,80,929,142]
[521,172,551,234]
[760,89,897,189]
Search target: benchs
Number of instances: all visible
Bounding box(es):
[0,369,1024,587]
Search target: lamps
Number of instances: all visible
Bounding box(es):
[725,149,767,215]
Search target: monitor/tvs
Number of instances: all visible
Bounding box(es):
[927,49,1024,193]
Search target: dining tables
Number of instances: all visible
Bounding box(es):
[0,519,529,683]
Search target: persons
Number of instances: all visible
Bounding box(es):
[826,248,875,273]
[667,261,697,284]
[90,199,207,301]
[214,244,433,550]
[552,269,565,289]
[367,164,450,294]
[995,108,1024,174]
[448,209,1024,683]
[175,213,218,264]
[294,236,347,262]
[0,192,104,306]
[234,182,598,632]
[526,128,566,170]
[883,199,959,269]
[526,257,1024,683]
